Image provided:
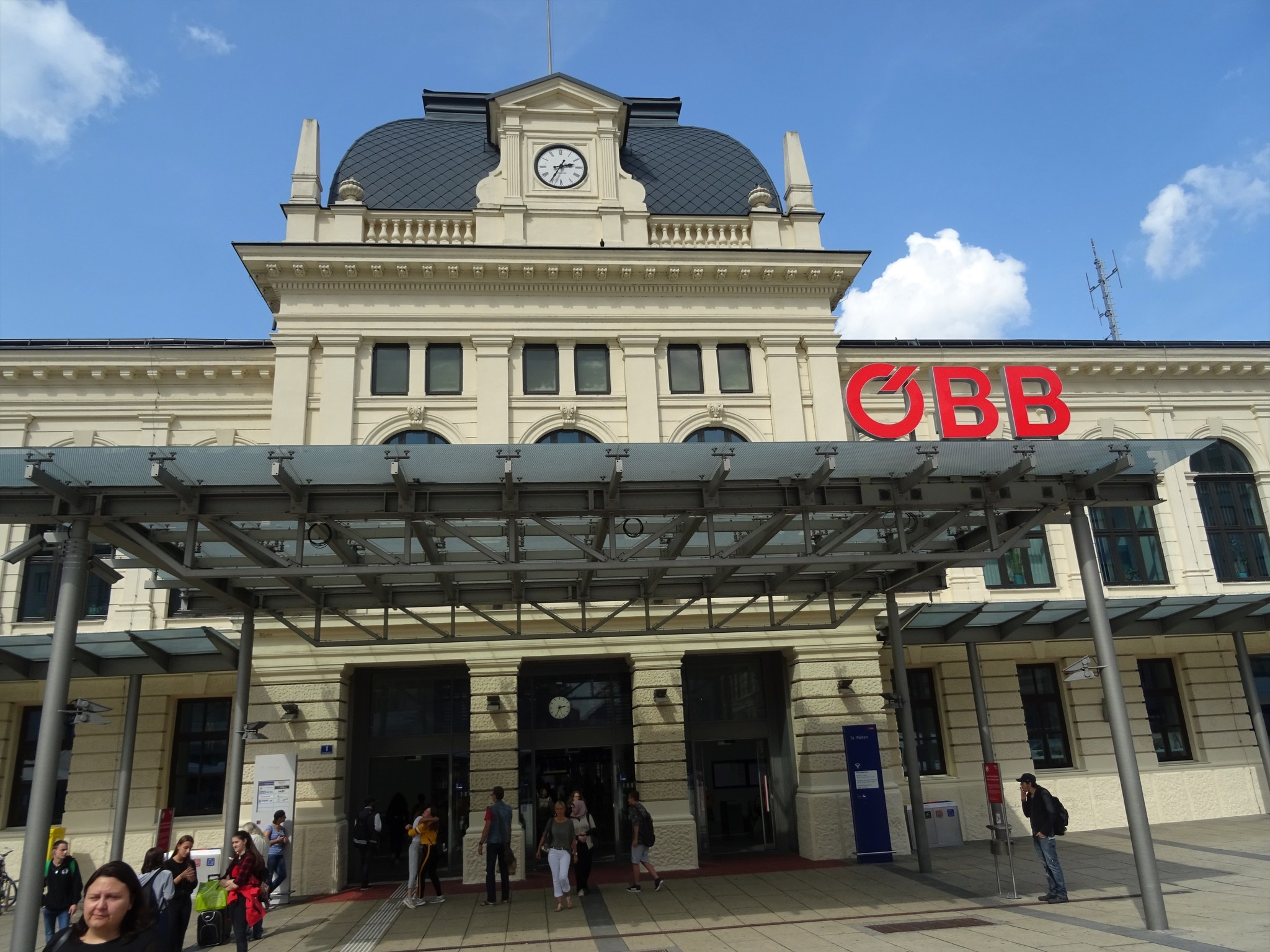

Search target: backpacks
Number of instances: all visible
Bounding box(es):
[1037,787,1069,836]
[354,810,376,840]
[140,868,168,927]
[630,805,655,847]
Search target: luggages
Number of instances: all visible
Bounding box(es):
[197,874,224,947]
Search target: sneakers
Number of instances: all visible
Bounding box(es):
[578,889,584,896]
[566,896,572,907]
[555,903,564,911]
[627,885,641,892]
[429,895,445,903]
[654,878,663,891]
[1048,895,1069,903]
[403,893,426,909]
[1038,893,1059,901]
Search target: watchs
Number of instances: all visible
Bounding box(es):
[574,852,577,855]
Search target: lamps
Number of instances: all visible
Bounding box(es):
[837,679,855,693]
[237,721,269,739]
[486,696,500,710]
[61,698,114,724]
[1062,655,1105,682]
[879,693,904,709]
[280,704,299,719]
[654,688,667,703]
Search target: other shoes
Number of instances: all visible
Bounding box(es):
[480,900,495,906]
[268,902,275,909]
[361,885,373,890]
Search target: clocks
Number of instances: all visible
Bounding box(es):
[549,696,570,719]
[534,145,588,189]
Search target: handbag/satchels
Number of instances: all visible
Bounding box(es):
[540,817,554,854]
[496,847,517,875]
[195,880,228,912]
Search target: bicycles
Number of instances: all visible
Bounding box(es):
[0,850,18,915]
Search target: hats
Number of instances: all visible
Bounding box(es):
[1016,773,1036,784]
[272,810,285,824]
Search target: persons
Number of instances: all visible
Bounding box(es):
[264,810,288,906]
[40,840,82,944]
[403,808,446,908]
[220,830,266,952]
[536,801,578,911]
[537,783,582,839]
[243,822,270,939]
[478,786,512,905]
[570,800,596,896]
[138,848,175,931]
[164,835,198,952]
[1016,773,1069,903]
[42,861,168,952]
[627,791,663,892]
[353,796,382,890]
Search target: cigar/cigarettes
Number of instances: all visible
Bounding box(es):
[1017,788,1022,790]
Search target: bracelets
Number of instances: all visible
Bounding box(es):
[276,839,278,844]
[479,842,483,845]
